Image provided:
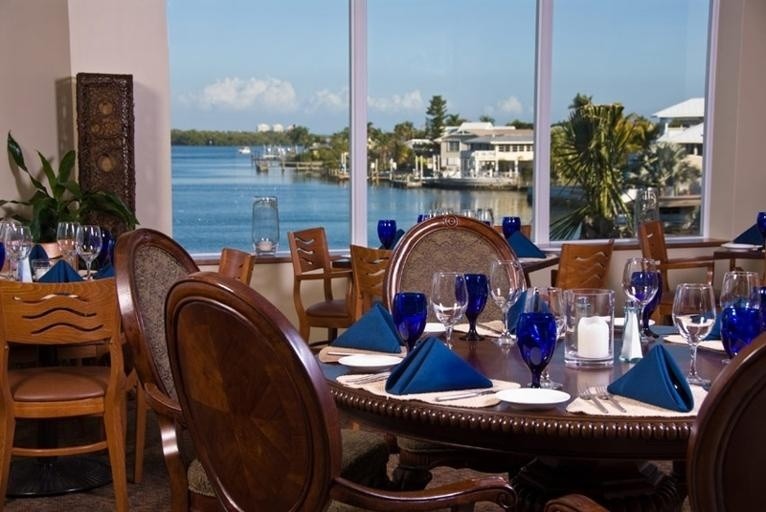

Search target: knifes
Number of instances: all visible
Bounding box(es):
[434,386,498,403]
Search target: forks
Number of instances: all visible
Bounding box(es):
[578,385,608,414]
[595,385,626,414]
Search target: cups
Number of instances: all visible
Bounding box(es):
[376,209,522,250]
[558,287,617,369]
[250,194,280,256]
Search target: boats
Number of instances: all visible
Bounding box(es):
[237,144,291,173]
[393,174,423,189]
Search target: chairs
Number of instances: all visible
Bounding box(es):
[286,209,763,509]
[165,273,518,510]
[1,210,254,511]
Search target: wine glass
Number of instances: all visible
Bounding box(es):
[456,275,489,340]
[490,261,524,346]
[393,292,426,356]
[0,220,103,281]
[718,272,755,365]
[433,273,471,348]
[671,282,712,388]
[610,191,658,238]
[622,257,657,343]
[514,285,561,388]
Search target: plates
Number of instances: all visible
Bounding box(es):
[721,242,760,251]
[339,355,405,370]
[494,389,573,412]
